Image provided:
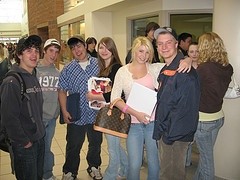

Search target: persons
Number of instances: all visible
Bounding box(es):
[36,38,61,180]
[0,35,46,180]
[56,35,103,180]
[110,34,192,180]
[0,22,201,168]
[192,31,233,180]
[154,28,201,180]
[85,37,128,180]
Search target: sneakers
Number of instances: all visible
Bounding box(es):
[87,165,102,179]
[61,170,77,180]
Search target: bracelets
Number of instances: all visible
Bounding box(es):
[123,105,129,113]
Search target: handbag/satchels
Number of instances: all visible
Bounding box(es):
[59,92,81,125]
[223,70,240,99]
[93,98,132,139]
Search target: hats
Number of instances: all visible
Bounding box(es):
[153,26,177,39]
[43,37,60,48]
[67,34,85,44]
[15,34,42,50]
